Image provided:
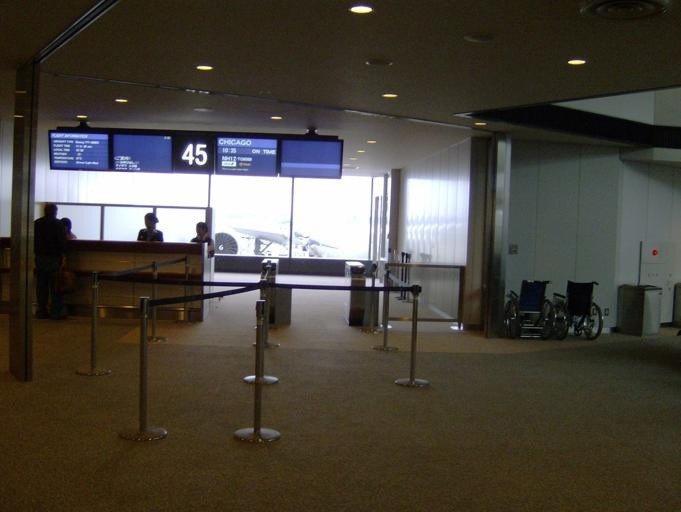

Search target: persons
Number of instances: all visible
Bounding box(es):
[61,216,76,239]
[190,219,215,258]
[33,202,69,320]
[136,212,163,241]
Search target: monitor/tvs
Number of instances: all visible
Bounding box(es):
[111,131,173,173]
[280,137,343,179]
[215,134,278,177]
[48,130,111,172]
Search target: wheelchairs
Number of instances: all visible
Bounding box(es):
[552,278,606,340]
[506,277,556,340]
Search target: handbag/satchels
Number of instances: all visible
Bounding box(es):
[51,255,77,295]
